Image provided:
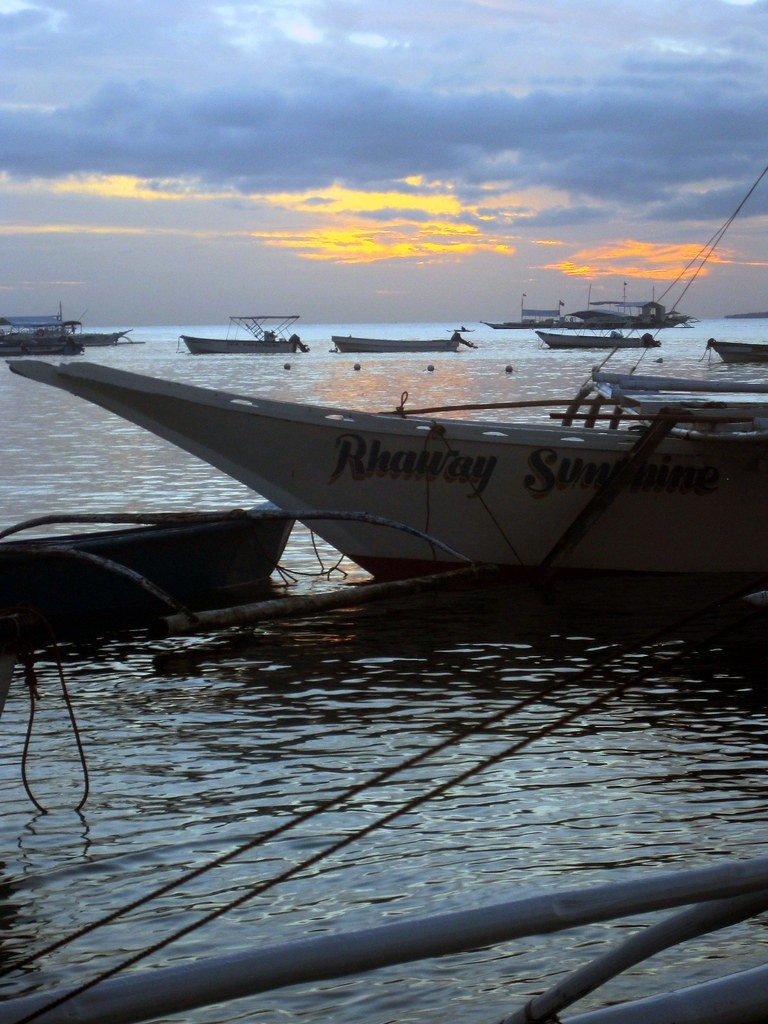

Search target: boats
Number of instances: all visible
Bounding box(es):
[177,315,312,354]
[6,163,768,590]
[534,325,661,349]
[699,338,767,363]
[1,501,295,633]
[331,326,478,353]
[480,283,702,329]
[0,304,134,357]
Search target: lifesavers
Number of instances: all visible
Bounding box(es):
[37,329,44,337]
